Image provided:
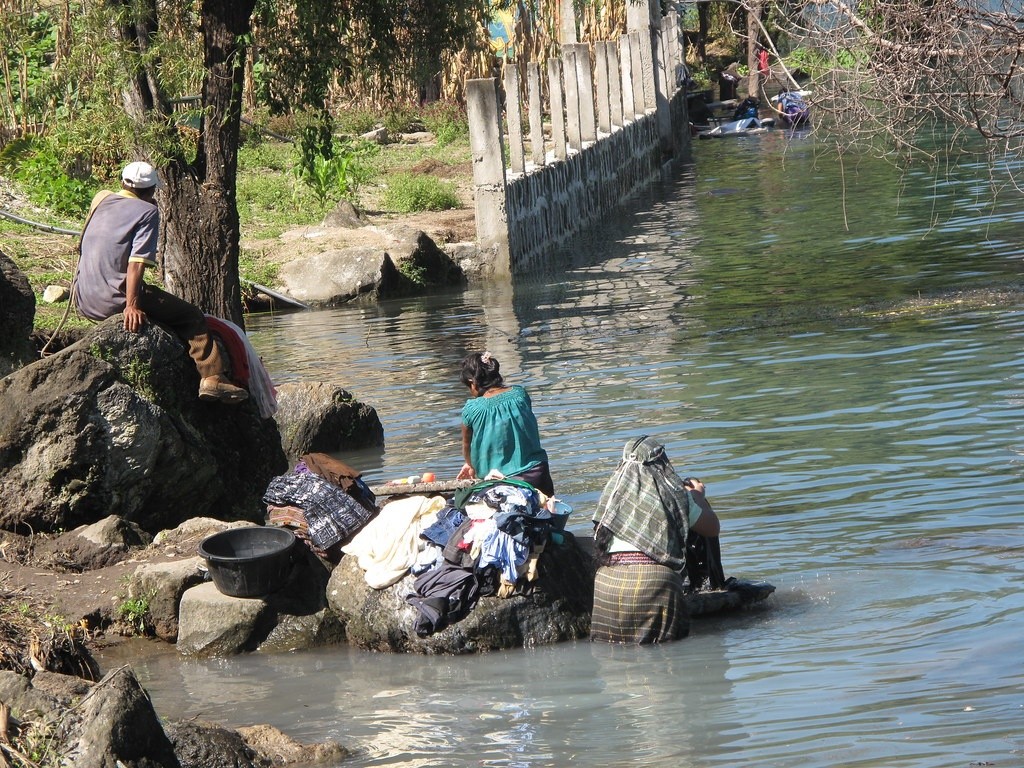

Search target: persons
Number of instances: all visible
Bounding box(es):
[457,350,555,500]
[777,88,809,124]
[589,435,721,647]
[70,162,251,406]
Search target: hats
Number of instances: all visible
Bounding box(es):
[124,161,164,190]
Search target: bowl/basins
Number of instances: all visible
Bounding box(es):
[198,526,297,598]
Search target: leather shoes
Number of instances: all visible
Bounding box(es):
[198,374,247,402]
